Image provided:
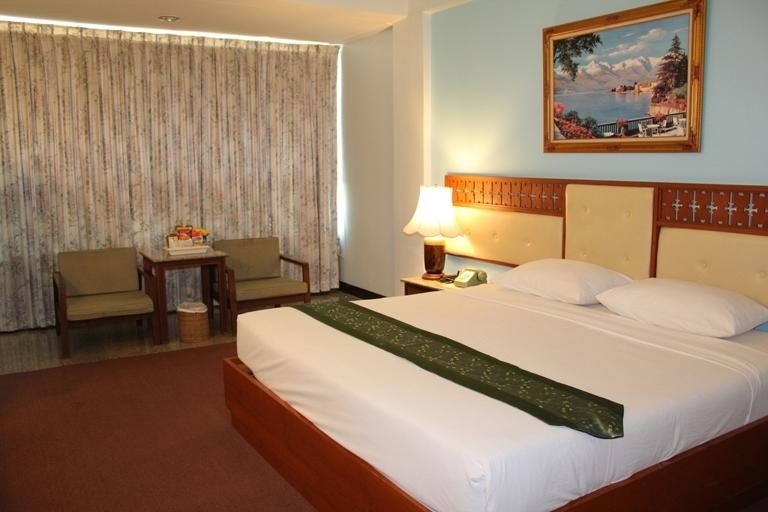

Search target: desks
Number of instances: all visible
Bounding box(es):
[137,244,231,345]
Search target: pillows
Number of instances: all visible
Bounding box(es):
[595,277,768,340]
[495,257,634,306]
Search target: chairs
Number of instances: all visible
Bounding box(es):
[51,269,164,360]
[208,252,311,336]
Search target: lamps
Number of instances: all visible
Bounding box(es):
[401,183,466,281]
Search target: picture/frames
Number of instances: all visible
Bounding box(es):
[541,0,707,155]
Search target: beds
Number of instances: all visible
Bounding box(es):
[222,283,767,511]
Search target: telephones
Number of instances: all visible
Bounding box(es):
[454,267,488,288]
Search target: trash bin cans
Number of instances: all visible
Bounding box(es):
[175,301,209,343]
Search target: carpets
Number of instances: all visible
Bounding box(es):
[0,337,318,511]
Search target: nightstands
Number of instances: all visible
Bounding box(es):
[401,274,467,295]
